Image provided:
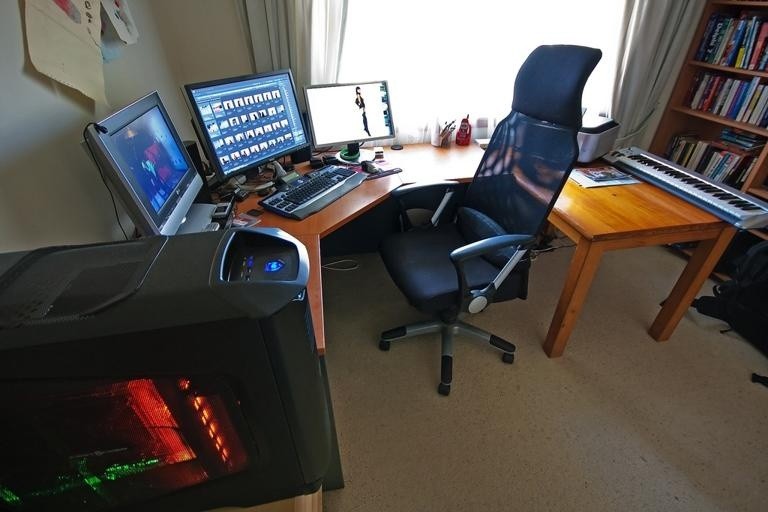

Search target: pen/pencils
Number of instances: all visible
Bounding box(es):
[439,120,456,147]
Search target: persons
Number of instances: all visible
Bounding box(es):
[354,86,372,137]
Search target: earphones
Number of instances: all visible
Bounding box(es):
[94,122,108,134]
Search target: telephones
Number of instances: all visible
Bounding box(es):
[456,114,471,146]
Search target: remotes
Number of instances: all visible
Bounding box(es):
[205,223,220,232]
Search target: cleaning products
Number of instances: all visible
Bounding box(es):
[455,117,472,146]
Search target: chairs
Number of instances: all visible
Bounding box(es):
[376,45,603,397]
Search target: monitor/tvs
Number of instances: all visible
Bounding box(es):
[303,79,395,166]
[180,68,311,187]
[85,89,216,236]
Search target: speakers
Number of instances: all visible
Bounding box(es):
[182,140,213,204]
[290,111,311,164]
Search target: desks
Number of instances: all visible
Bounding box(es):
[211,150,401,352]
[540,164,736,359]
[364,139,512,214]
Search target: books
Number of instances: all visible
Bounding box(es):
[571,166,641,190]
[662,10,767,193]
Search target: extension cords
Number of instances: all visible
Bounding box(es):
[205,172,215,182]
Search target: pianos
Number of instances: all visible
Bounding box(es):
[602,146,768,231]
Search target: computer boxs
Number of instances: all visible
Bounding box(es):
[0,226,333,512]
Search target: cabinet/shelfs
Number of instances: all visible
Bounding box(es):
[647,2,768,281]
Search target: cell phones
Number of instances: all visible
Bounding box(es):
[211,203,231,219]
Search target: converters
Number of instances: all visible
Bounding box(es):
[323,157,337,165]
[310,160,323,169]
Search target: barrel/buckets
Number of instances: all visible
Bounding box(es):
[399,208,440,230]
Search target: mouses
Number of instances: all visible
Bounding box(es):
[361,161,379,173]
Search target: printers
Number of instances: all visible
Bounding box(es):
[575,107,622,166]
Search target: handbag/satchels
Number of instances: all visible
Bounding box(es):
[696,242,768,358]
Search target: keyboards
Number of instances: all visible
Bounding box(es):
[258,165,367,221]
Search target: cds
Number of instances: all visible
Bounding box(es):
[232,213,257,227]
